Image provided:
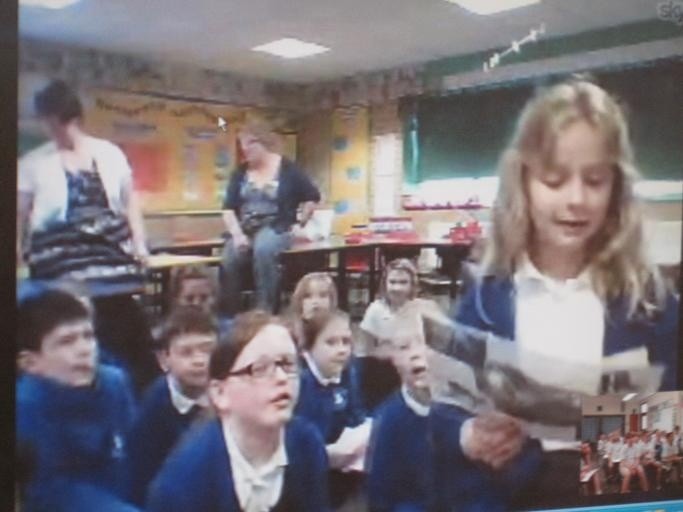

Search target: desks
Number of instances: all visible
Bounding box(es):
[17,238,487,356]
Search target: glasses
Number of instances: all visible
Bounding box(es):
[226,355,304,382]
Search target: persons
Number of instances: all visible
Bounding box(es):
[15,238,680,510]
[214,118,322,317]
[423,78,683,512]
[16,77,161,395]
